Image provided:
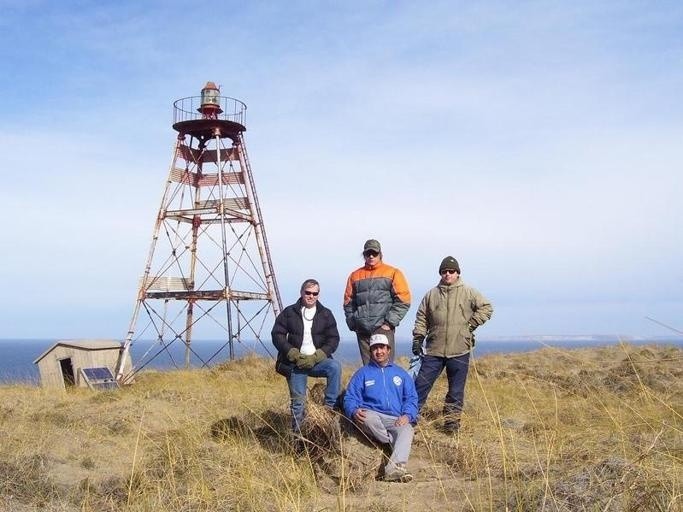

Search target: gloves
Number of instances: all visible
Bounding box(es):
[412,336,426,358]
[468,321,477,334]
[286,347,327,370]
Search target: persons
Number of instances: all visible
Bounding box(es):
[341,333,419,482]
[343,238,410,367]
[412,256,492,434]
[270,278,342,453]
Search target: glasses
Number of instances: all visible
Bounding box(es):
[364,250,380,257]
[441,269,457,274]
[304,290,319,296]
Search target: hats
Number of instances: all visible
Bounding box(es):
[439,256,460,274]
[369,334,389,348]
[364,239,381,253]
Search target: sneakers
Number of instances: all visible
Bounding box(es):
[383,471,413,483]
[384,441,394,455]
